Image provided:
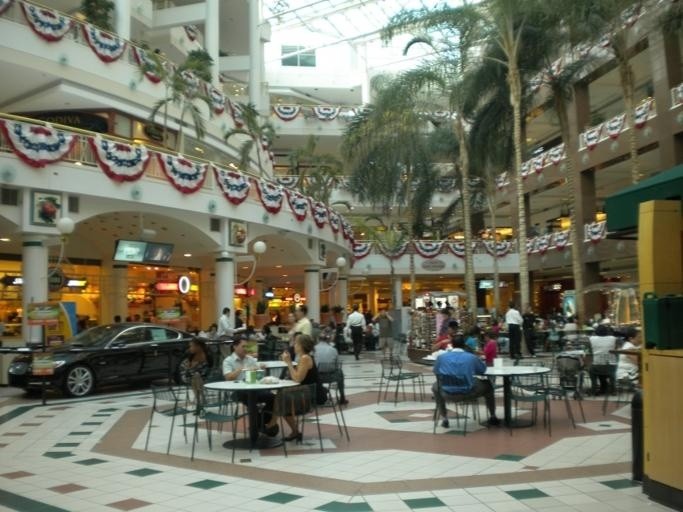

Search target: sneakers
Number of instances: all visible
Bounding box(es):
[441,420,449,427]
[356,354,360,359]
[340,399,349,405]
[489,417,499,425]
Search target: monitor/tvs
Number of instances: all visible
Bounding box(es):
[112,240,148,262]
[143,242,175,264]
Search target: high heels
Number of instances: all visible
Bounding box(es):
[281,433,302,443]
[257,425,279,437]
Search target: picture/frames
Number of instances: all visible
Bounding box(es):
[228,219,249,247]
[30,191,63,227]
[319,239,327,261]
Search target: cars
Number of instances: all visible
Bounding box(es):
[6,319,215,403]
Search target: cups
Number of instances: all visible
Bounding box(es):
[240,369,264,384]
[492,357,504,370]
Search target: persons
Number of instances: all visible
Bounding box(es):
[77,315,90,334]
[425,301,642,427]
[173,298,183,314]
[113,309,155,325]
[186,302,395,450]
[1,312,17,347]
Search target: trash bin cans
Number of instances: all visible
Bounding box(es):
[631,391,643,484]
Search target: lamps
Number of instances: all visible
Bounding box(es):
[234,240,266,286]
[43,218,75,280]
[320,257,346,291]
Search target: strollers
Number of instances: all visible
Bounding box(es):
[552,349,591,402]
